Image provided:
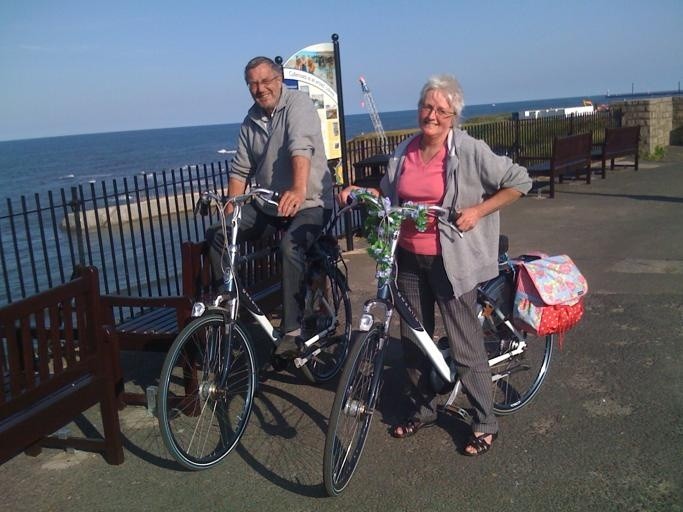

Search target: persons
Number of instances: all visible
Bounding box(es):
[196,56,334,367]
[334,73,533,457]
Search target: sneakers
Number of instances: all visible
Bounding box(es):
[273,334,303,359]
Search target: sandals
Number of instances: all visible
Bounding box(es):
[464,432,496,456]
[391,417,436,438]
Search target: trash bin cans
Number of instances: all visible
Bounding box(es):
[353,154,394,238]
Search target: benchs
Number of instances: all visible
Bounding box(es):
[100,229,327,417]
[1,263,123,469]
[588,123,642,178]
[516,127,593,197]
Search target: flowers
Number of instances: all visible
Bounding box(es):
[347,184,429,284]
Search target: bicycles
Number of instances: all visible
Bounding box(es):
[321,189,554,499]
[156,189,353,470]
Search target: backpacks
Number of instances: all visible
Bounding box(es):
[508,252,588,338]
